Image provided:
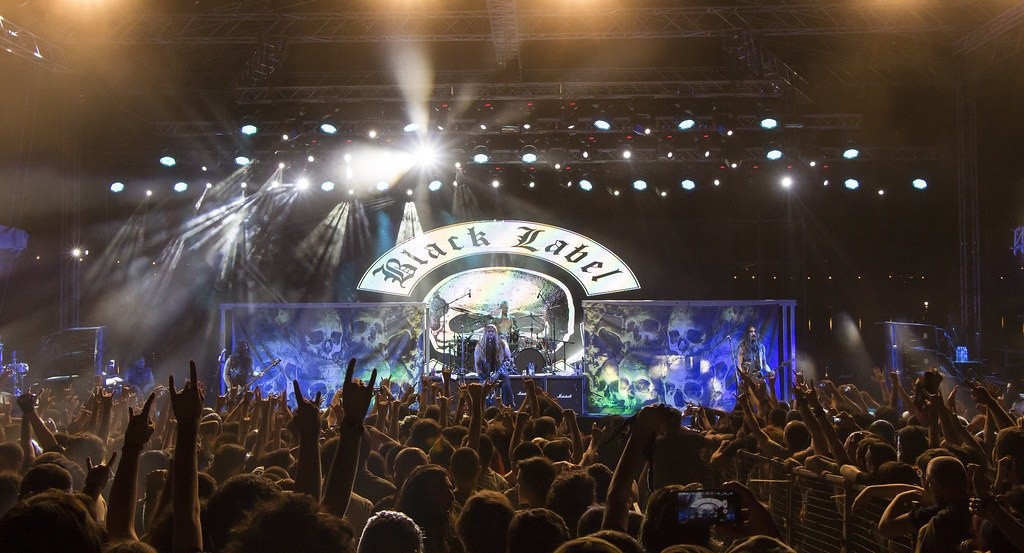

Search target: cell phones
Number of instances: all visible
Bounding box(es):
[672,489,741,528]
[844,387,850,392]
[832,416,842,423]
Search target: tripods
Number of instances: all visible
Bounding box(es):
[817,382,825,388]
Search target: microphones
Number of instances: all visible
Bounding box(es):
[469,289,471,298]
[537,291,541,298]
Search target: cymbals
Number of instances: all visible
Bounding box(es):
[451,307,471,313]
[519,314,545,317]
[468,313,490,320]
[520,326,541,332]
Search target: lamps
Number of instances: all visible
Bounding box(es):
[157,108,860,193]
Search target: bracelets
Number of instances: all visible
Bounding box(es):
[122,445,142,451]
[815,410,825,418]
[981,495,995,509]
[761,398,770,405]
[989,400,998,410]
[813,406,823,413]
[340,421,364,434]
[990,483,1003,494]
[955,426,965,434]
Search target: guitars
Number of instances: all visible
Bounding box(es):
[480,348,519,400]
[220,358,281,404]
[750,359,793,396]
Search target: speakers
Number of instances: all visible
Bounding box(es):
[882,321,940,414]
[462,376,587,416]
[63,326,105,376]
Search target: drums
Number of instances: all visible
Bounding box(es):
[515,348,543,374]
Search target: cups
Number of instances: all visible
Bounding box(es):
[430,368,436,376]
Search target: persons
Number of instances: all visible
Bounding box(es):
[736,324,776,404]
[474,324,516,408]
[223,341,264,393]
[490,300,520,358]
[0,362,1024,553]
[123,354,156,402]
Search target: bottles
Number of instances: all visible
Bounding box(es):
[579,363,582,374]
[956,346,968,362]
[528,363,535,376]
[573,363,577,376]
